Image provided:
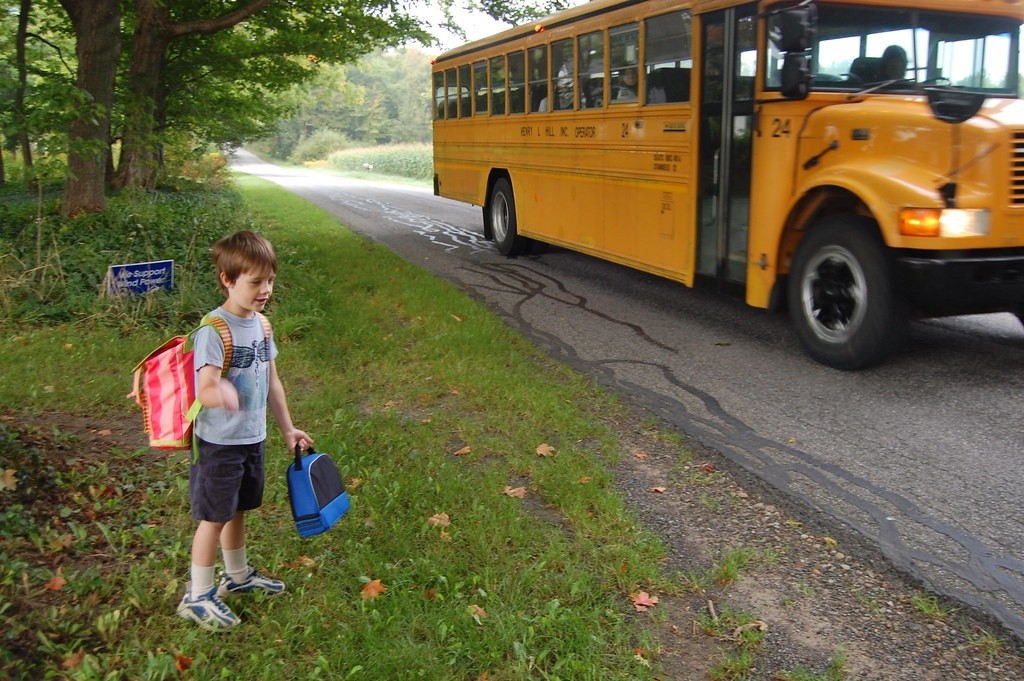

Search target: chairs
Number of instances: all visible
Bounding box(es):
[438,68,692,120]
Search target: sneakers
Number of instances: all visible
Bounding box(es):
[219,566,285,599]
[177,581,242,632]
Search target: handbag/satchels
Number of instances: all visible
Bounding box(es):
[286,443,351,539]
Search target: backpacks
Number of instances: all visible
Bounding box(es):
[126,312,271,465]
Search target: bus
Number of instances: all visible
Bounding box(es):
[430,1,1023,368]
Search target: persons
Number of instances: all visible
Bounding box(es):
[865,45,922,89]
[534,61,667,113]
[178,232,315,633]
